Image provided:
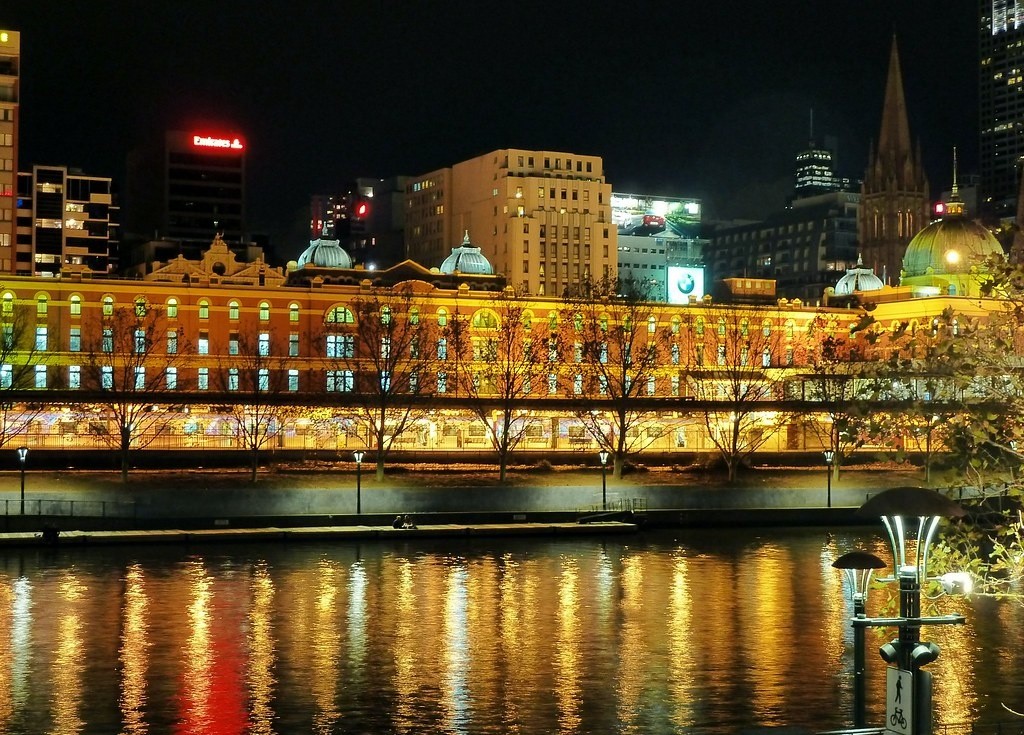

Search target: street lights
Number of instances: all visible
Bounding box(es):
[352,449,366,513]
[1011,440,1018,488]
[833,550,887,731]
[17,447,28,518]
[859,489,973,735]
[823,450,835,508]
[597,449,611,508]
